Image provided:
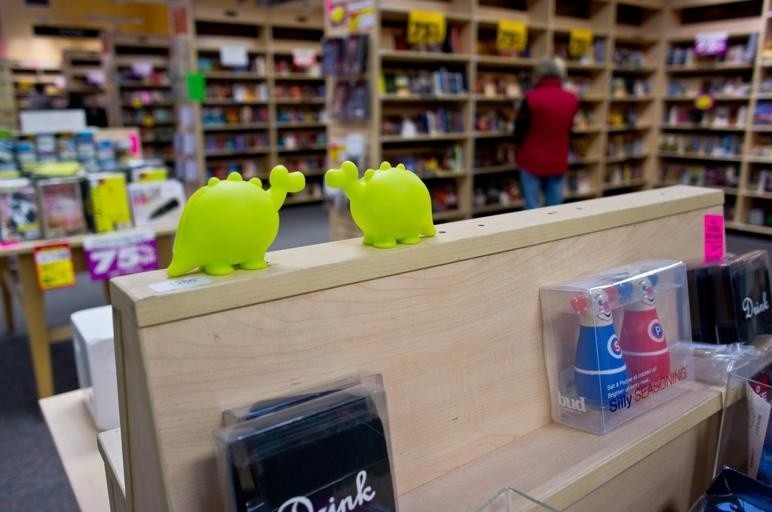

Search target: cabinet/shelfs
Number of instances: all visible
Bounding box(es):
[33,182,771,511]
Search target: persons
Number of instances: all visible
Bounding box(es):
[25,81,53,110]
[512,52,579,209]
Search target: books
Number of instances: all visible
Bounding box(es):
[378,28,535,215]
[554,29,771,225]
[199,38,326,202]
[1,161,182,238]
[119,67,175,163]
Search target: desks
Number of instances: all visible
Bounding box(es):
[1,226,180,403]
